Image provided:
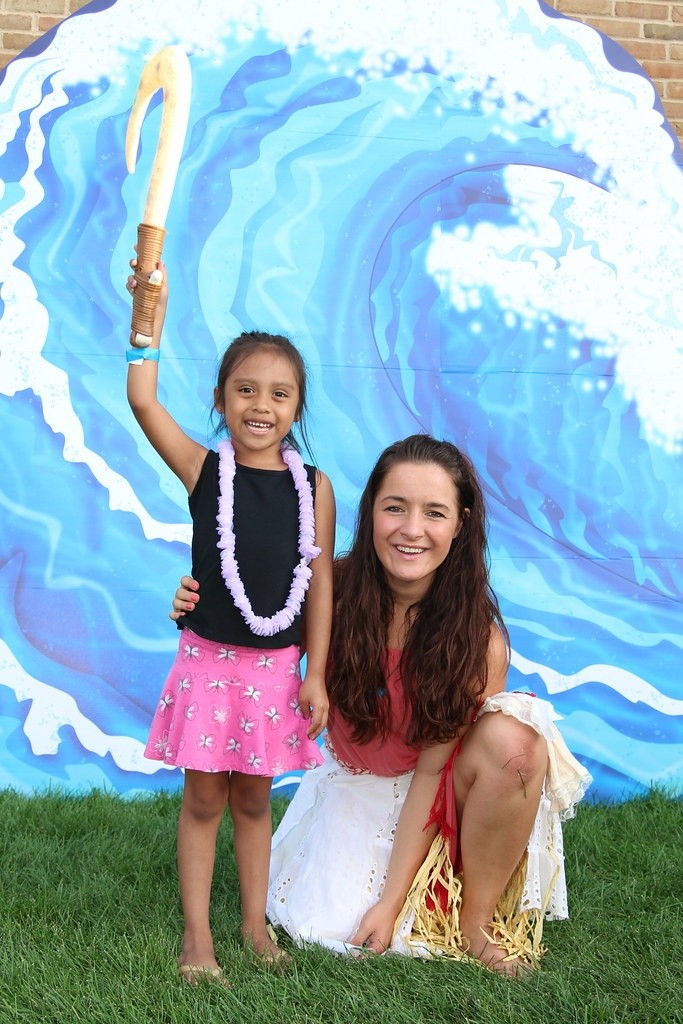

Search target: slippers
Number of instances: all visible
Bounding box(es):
[177,965,232,992]
[240,950,293,976]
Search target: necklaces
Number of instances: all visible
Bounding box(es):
[216,438,322,638]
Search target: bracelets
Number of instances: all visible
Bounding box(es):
[127,348,160,364]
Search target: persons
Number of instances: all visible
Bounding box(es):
[125,247,336,988]
[168,435,594,976]
